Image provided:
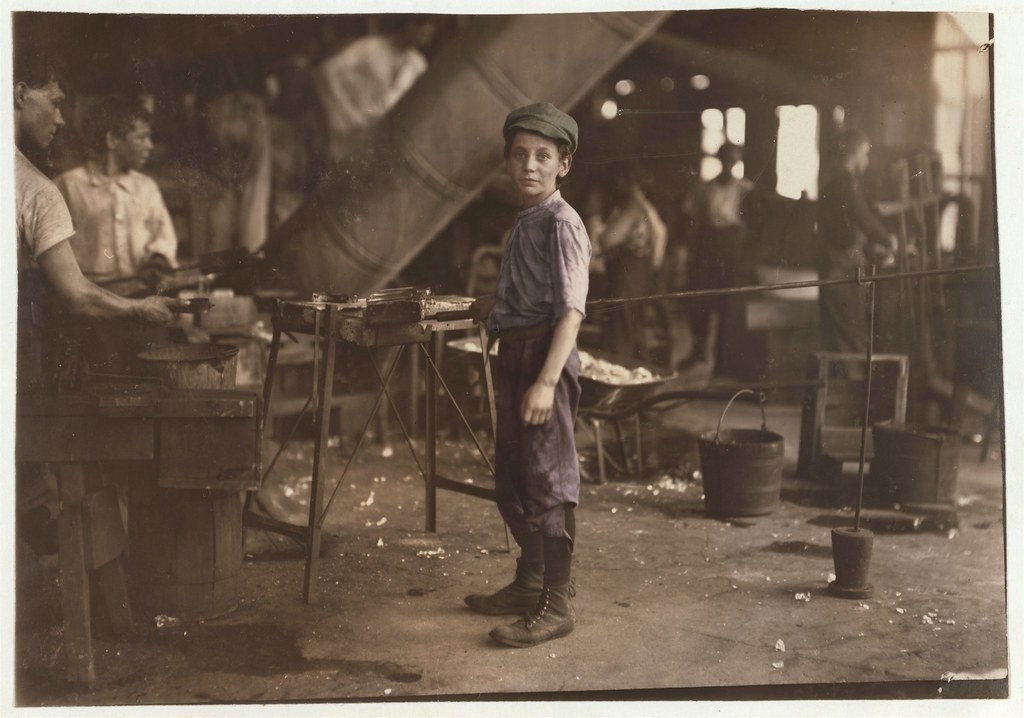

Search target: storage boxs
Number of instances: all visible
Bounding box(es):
[157,388,262,492]
[128,479,242,621]
[17,371,160,461]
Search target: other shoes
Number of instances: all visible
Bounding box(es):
[677,353,708,373]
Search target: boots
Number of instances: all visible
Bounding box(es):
[464,558,576,648]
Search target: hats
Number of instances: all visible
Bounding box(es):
[502,102,578,156]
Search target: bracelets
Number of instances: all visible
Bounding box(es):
[538,374,558,387]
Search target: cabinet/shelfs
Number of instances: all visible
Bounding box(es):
[795,347,908,477]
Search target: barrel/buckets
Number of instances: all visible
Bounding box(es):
[697,389,786,519]
[874,421,963,521]
[138,343,240,390]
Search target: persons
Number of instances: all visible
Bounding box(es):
[14,52,191,324]
[463,102,593,650]
[679,143,762,364]
[814,127,899,354]
[583,174,675,367]
[51,99,179,286]
